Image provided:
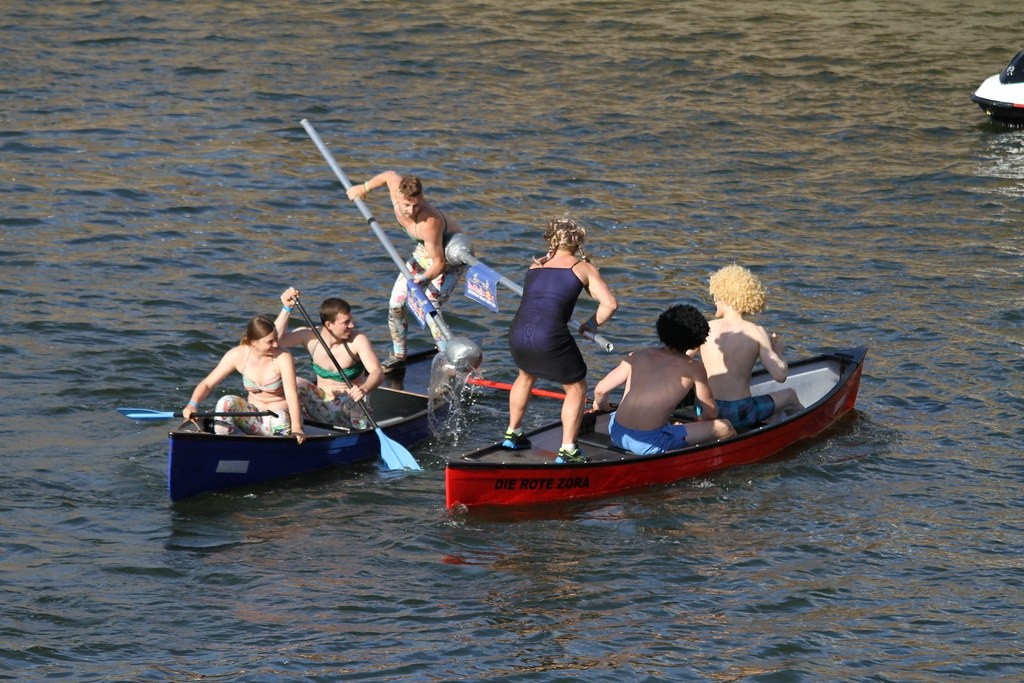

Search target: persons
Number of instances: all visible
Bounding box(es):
[500,215,618,463]
[274,287,386,430]
[592,305,738,454]
[686,264,806,427]
[182,315,306,444]
[346,169,474,373]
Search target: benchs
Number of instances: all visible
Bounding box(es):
[675,405,768,434]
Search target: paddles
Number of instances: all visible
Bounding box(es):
[288,289,422,470]
[116,406,280,421]
[464,376,700,422]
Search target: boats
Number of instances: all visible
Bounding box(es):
[168,348,449,502]
[970,49,1024,120]
[445,343,868,509]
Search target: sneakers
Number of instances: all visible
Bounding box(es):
[503,431,532,449]
[380,352,407,373]
[554,445,592,463]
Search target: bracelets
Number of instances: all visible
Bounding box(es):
[418,275,428,283]
[364,182,371,192]
[588,319,597,329]
[188,401,199,408]
[283,305,294,312]
